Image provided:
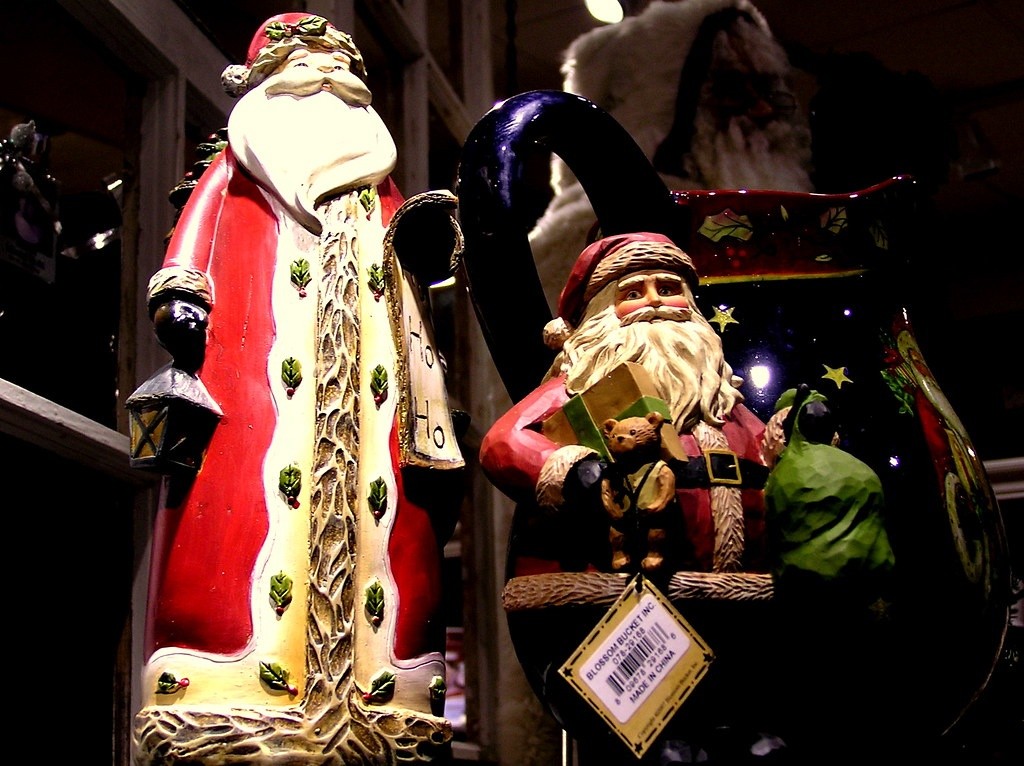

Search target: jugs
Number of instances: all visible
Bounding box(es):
[457,90,1014,766]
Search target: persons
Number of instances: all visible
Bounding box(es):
[480,233,895,577]
[147,14,466,764]
